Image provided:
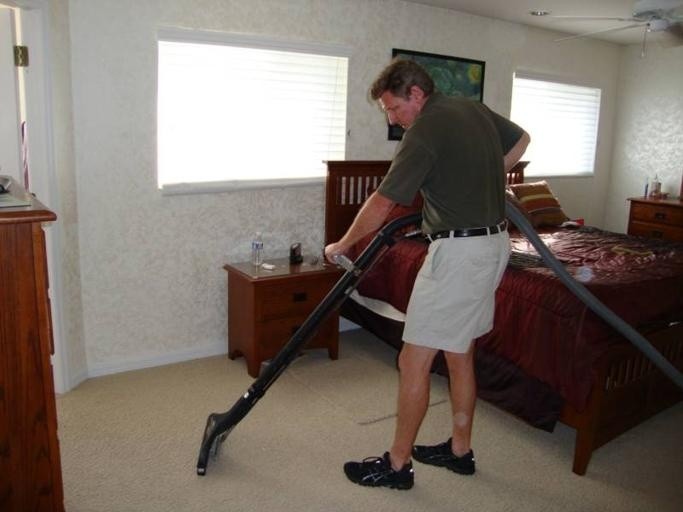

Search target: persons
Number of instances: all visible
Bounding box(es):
[322,54,533,492]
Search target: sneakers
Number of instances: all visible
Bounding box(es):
[412,437,475,474]
[344,452,413,490]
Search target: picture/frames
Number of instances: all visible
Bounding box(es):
[387,46,487,141]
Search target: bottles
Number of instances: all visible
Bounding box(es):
[252,231,264,266]
[641,175,649,197]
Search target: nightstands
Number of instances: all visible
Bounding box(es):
[222,255,347,378]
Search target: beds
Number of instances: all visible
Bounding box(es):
[321,158,683,477]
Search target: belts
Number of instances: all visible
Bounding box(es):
[425,223,506,245]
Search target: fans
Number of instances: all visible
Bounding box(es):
[545,2,683,58]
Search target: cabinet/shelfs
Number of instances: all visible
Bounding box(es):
[627,197,683,244]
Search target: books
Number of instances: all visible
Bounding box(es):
[0,192,31,213]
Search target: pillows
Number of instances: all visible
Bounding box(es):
[367,187,424,233]
[505,178,570,229]
